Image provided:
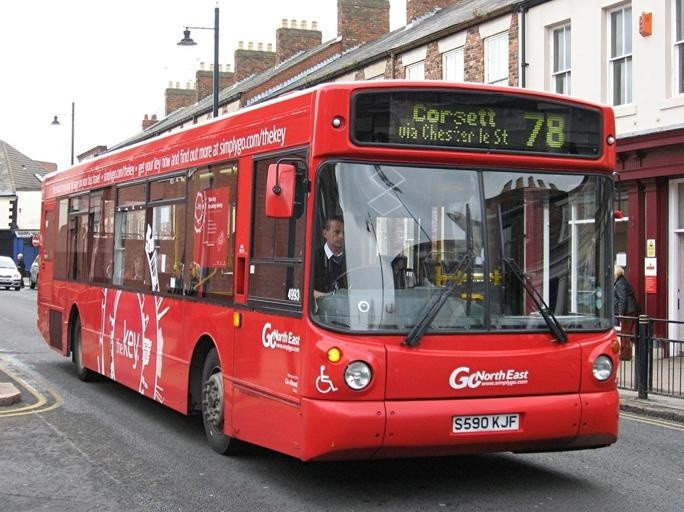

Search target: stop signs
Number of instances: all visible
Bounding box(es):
[32,236,40,247]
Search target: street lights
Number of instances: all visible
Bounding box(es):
[176,8,219,117]
[51,103,74,166]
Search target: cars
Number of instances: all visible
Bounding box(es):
[29,255,40,289]
[0,254,22,290]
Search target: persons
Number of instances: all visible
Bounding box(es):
[17,253,25,288]
[312,216,348,298]
[613,265,641,360]
[125,256,144,281]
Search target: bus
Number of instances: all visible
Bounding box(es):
[38,80,619,462]
